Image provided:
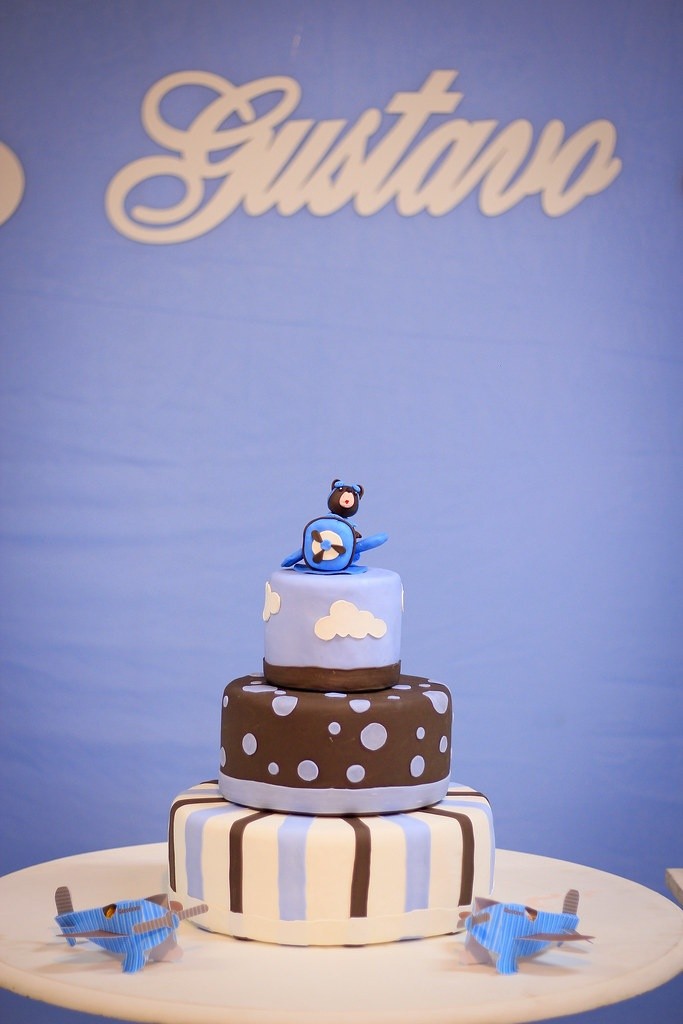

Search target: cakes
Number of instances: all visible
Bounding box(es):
[166,565,496,944]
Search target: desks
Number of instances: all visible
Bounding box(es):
[0,843,682,1024]
